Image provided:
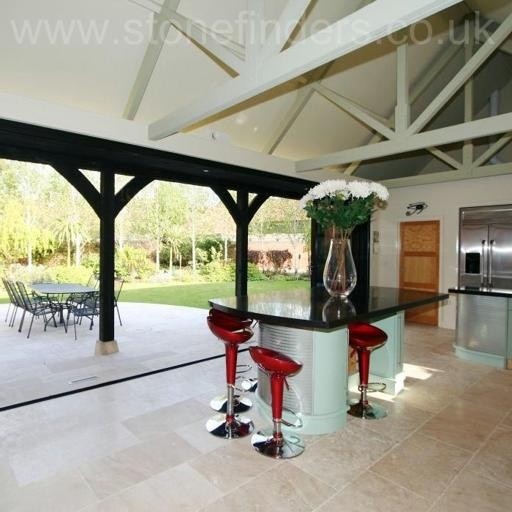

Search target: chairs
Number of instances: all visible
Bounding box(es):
[1,272,125,340]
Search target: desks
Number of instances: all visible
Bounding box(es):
[207,287,450,435]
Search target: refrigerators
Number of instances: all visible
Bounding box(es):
[457,203,512,288]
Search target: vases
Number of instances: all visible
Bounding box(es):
[322,237,359,298]
[322,296,357,323]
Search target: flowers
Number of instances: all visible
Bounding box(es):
[301,178,391,289]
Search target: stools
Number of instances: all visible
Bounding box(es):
[209,307,252,412]
[207,316,255,439]
[347,324,388,419]
[249,346,306,459]
